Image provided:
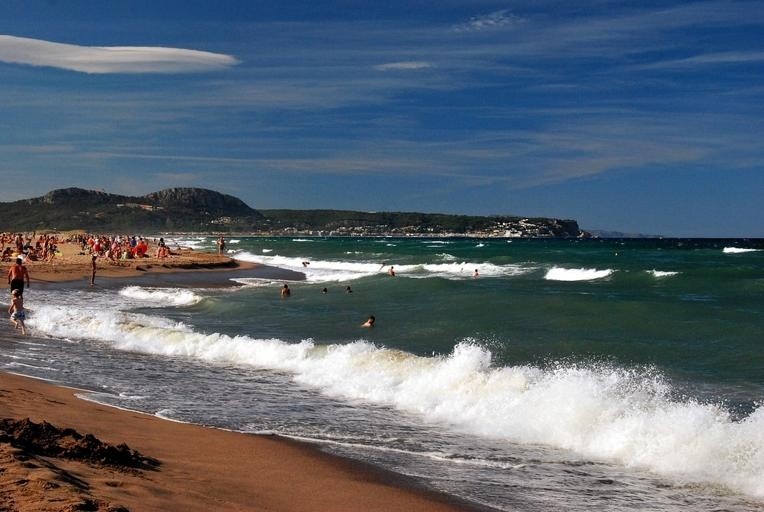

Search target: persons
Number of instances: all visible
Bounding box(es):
[0,228,174,267]
[8,289,26,335]
[473,269,478,277]
[88,255,98,286]
[361,315,375,328]
[7,257,29,299]
[346,286,352,294]
[302,261,310,267]
[387,266,395,276]
[281,284,291,297]
[217,236,225,254]
